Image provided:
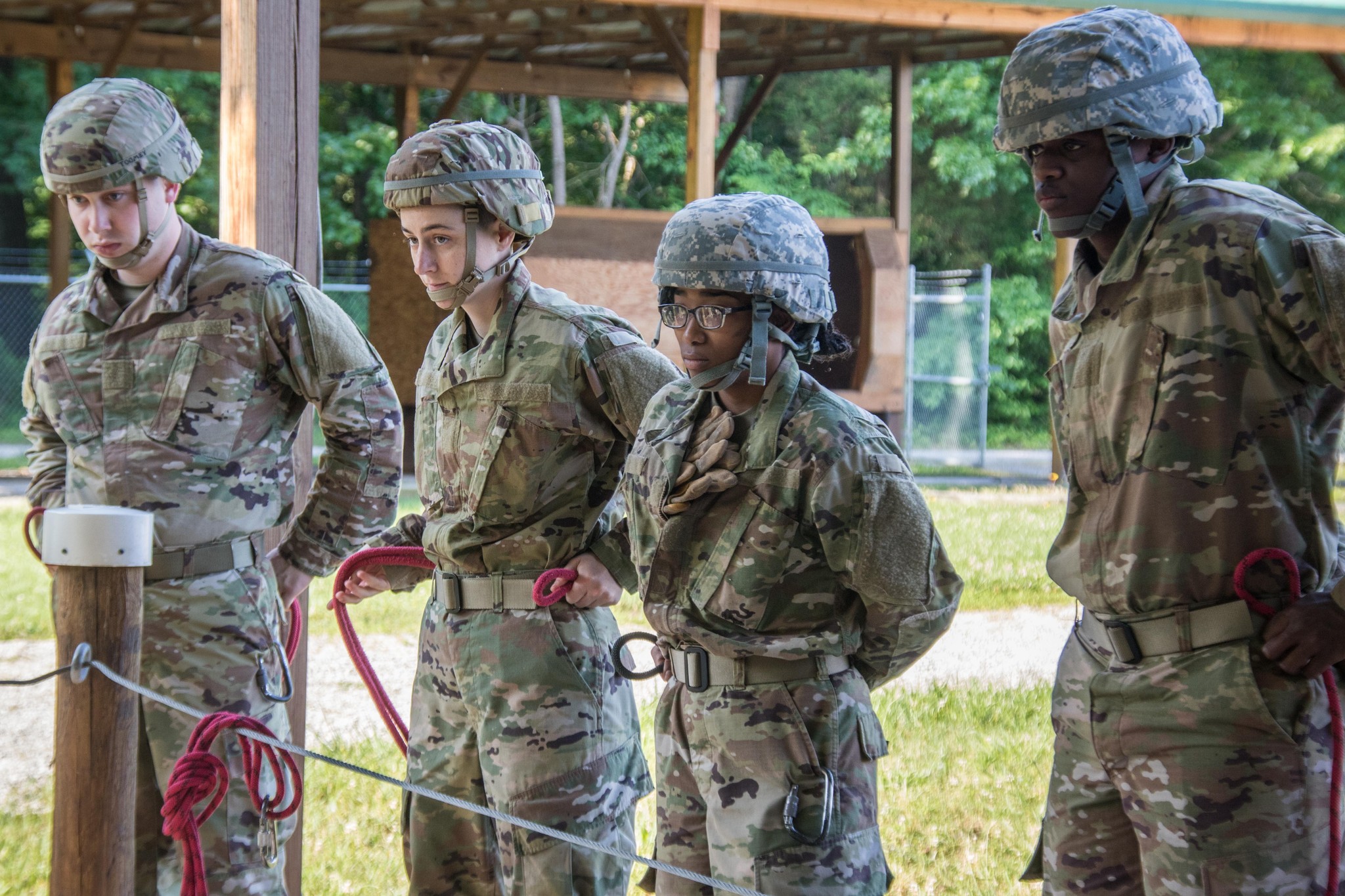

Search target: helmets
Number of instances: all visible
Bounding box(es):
[650,191,839,324]
[384,117,554,236]
[994,3,1224,152]
[39,77,206,195]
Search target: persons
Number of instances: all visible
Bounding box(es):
[21,77,406,895]
[326,117,684,896]
[623,192,964,896]
[989,4,1345,896]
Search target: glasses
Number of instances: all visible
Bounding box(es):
[658,304,749,329]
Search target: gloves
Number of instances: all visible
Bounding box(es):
[663,405,742,515]
